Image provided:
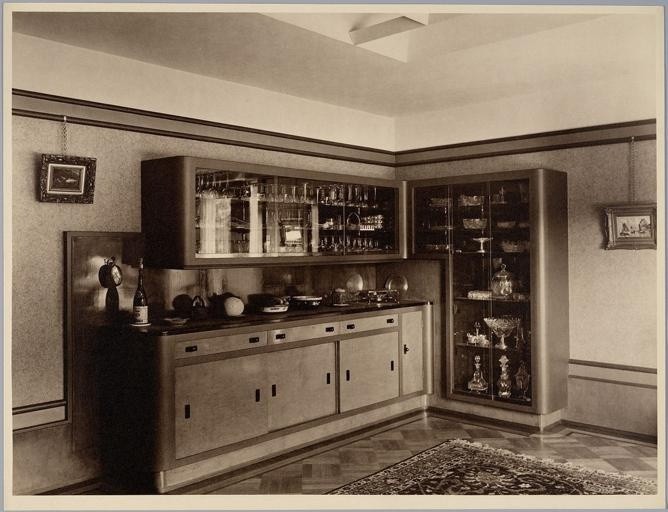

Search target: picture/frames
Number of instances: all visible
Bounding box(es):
[600,203,656,250]
[38,153,97,205]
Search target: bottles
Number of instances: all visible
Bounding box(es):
[132,257,149,323]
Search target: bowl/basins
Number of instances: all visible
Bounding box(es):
[361,289,398,302]
[497,221,516,228]
[292,295,323,310]
[463,218,488,230]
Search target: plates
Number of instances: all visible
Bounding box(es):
[164,318,192,326]
[385,273,408,297]
[130,323,152,327]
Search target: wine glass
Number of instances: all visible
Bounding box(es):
[319,232,379,254]
[196,170,378,209]
[484,314,521,350]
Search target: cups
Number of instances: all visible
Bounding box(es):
[234,239,248,253]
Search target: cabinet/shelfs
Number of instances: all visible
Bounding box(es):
[176,333,268,461]
[339,314,400,415]
[401,311,424,395]
[182,156,407,266]
[452,181,534,409]
[409,181,449,257]
[268,322,339,433]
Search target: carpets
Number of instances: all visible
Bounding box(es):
[322,437,658,495]
